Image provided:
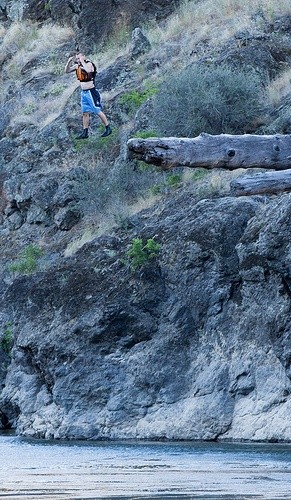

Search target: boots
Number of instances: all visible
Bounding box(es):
[75,128,89,139]
[102,124,113,137]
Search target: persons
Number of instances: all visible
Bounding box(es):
[64,51,112,140]
